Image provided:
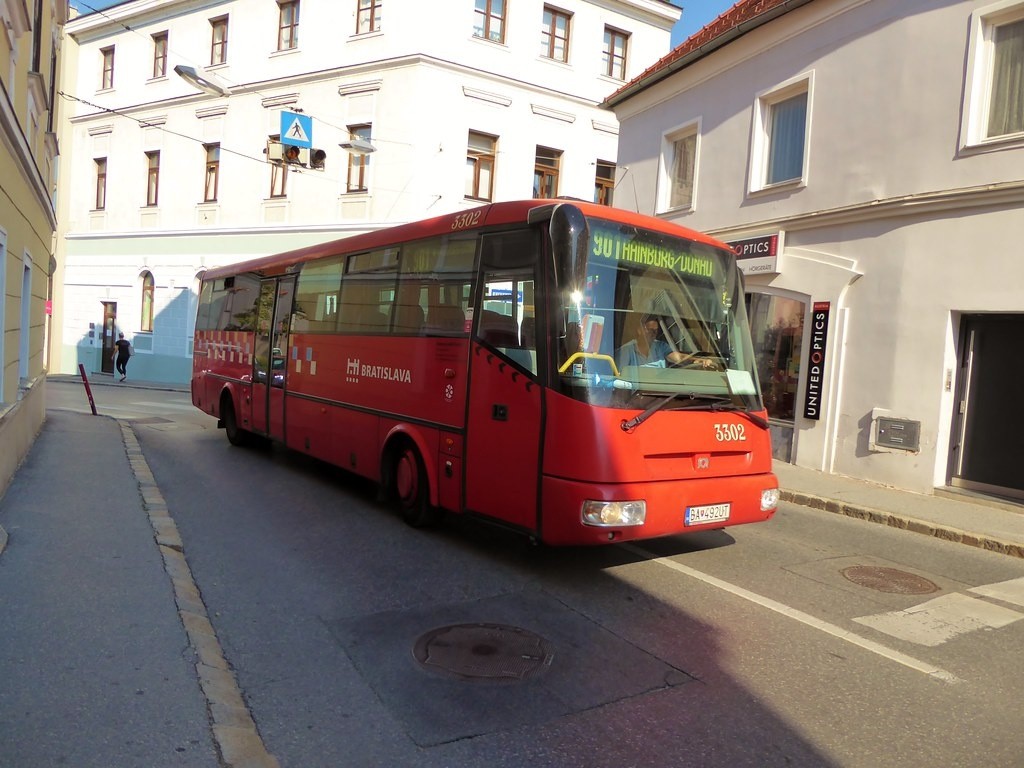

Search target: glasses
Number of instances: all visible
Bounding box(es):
[644,328,659,335]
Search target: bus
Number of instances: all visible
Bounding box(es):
[199,344,284,385]
[190,195,781,548]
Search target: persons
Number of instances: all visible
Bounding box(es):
[111,333,130,382]
[614,313,712,373]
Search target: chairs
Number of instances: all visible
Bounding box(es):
[305,300,536,352]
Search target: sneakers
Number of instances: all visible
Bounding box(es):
[120,376,126,382]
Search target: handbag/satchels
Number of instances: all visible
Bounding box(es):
[127,345,135,356]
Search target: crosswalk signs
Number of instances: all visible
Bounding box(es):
[281,110,312,151]
